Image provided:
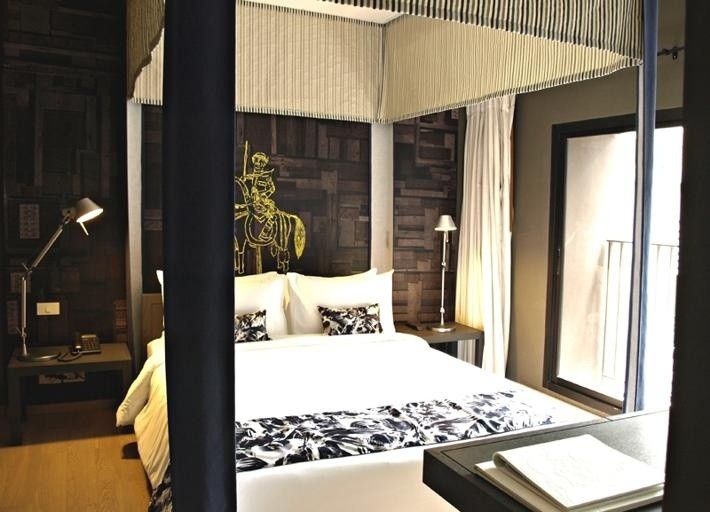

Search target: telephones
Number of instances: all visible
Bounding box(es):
[71,331,101,355]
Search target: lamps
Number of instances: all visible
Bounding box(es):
[14,198,104,363]
[431,214,457,333]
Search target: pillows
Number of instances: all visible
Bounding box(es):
[154,268,396,343]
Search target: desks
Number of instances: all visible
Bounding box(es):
[5,343,133,447]
[423,407,670,512]
[392,322,484,368]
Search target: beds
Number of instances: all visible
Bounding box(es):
[114,290,659,512]
